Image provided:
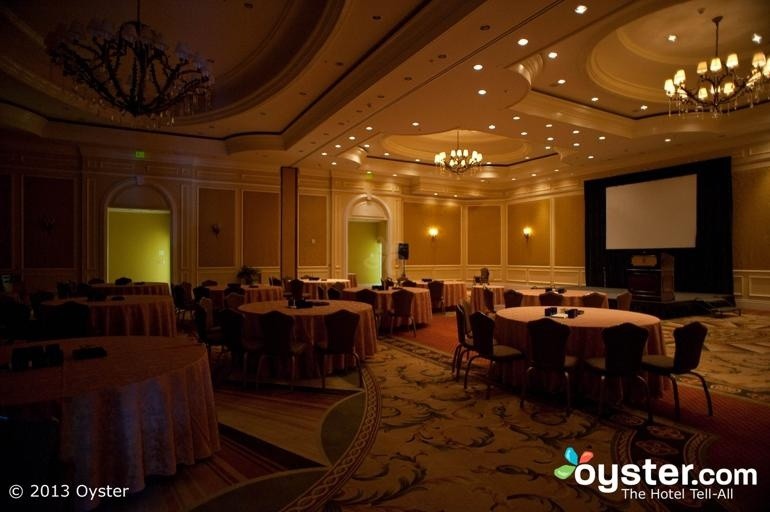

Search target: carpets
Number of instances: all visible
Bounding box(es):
[652,309,770,409]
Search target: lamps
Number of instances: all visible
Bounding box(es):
[398,242,409,278]
[431,128,487,175]
[664,13,765,123]
[44,0,214,131]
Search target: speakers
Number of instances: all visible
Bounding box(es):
[399,243,408,259]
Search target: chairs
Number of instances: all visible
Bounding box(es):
[473,268,490,285]
[0,282,715,512]
